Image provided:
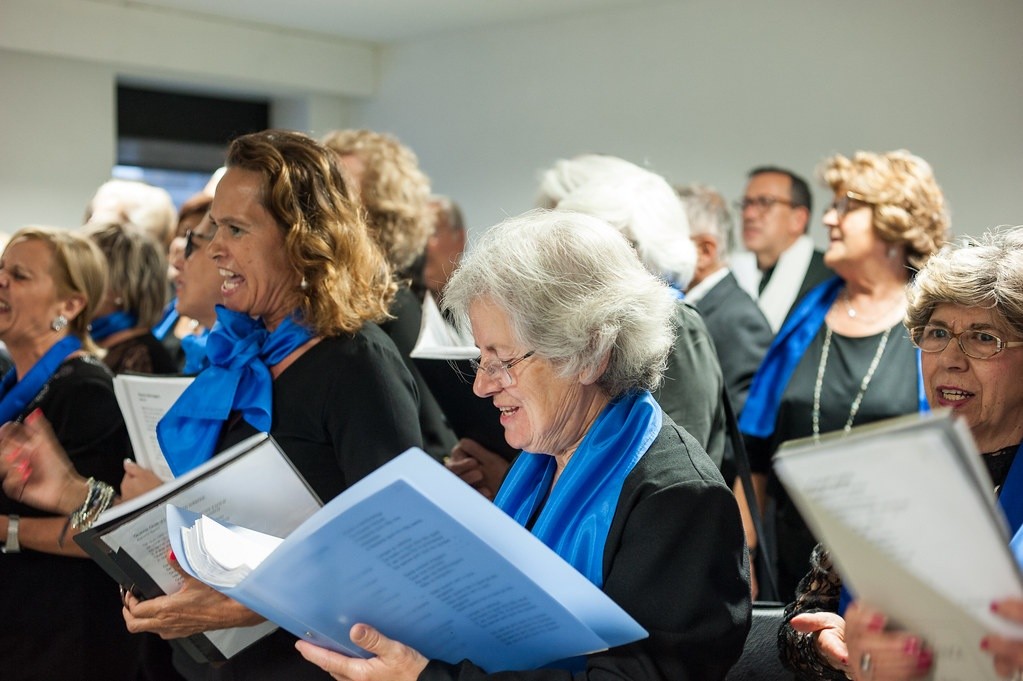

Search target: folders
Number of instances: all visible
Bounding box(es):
[71,431,325,666]
[165,444,653,669]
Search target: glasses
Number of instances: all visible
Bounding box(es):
[910,325,1023,360]
[731,194,797,213]
[184,229,213,258]
[469,350,535,385]
[822,191,876,216]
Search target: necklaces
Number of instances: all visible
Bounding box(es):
[844,292,899,323]
[811,288,903,439]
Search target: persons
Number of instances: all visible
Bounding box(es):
[0,127,1022,681]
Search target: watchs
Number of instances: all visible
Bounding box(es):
[2,515,20,554]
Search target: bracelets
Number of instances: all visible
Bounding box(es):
[859,652,869,672]
[68,476,114,532]
